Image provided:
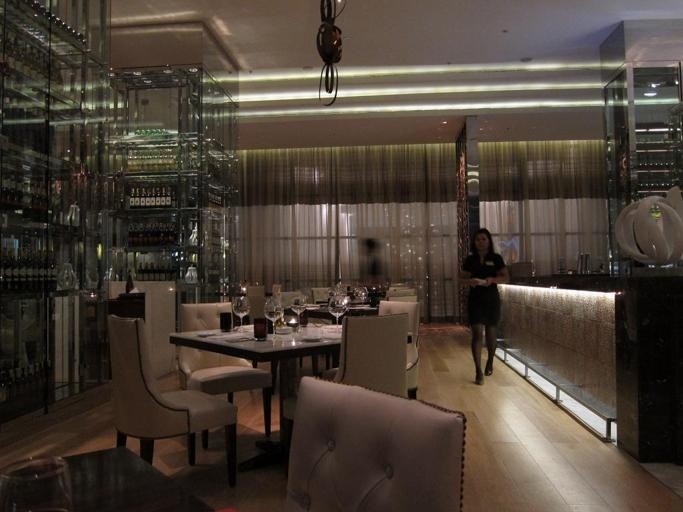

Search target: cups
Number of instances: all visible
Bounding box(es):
[217,312,232,332]
[557,256,568,273]
[252,316,266,341]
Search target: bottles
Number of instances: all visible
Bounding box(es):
[206,187,222,205]
[608,240,632,278]
[0,1,86,97]
[0,175,58,293]
[207,264,219,282]
[58,262,77,292]
[107,128,185,283]
[210,229,221,245]
[576,251,590,275]
[637,161,679,190]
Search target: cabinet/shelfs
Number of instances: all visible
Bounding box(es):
[0,0,110,293]
[110,133,199,282]
[636,140,683,199]
[199,138,238,283]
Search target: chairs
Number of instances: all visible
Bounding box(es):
[284,312,408,478]
[246,284,423,324]
[377,299,420,402]
[285,376,467,510]
[178,300,274,450]
[108,314,239,488]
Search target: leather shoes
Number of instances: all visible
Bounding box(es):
[476,373,483,385]
[485,360,492,376]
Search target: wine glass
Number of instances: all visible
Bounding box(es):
[263,295,285,340]
[327,293,348,340]
[348,278,389,306]
[230,296,256,335]
[287,293,310,332]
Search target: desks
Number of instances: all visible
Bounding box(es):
[0,447,214,512]
[169,322,413,455]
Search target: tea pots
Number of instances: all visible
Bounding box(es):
[183,267,197,285]
[186,250,199,263]
[187,221,204,248]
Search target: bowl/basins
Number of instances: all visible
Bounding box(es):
[295,323,324,341]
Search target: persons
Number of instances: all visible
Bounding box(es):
[357,236,386,283]
[456,227,510,384]
